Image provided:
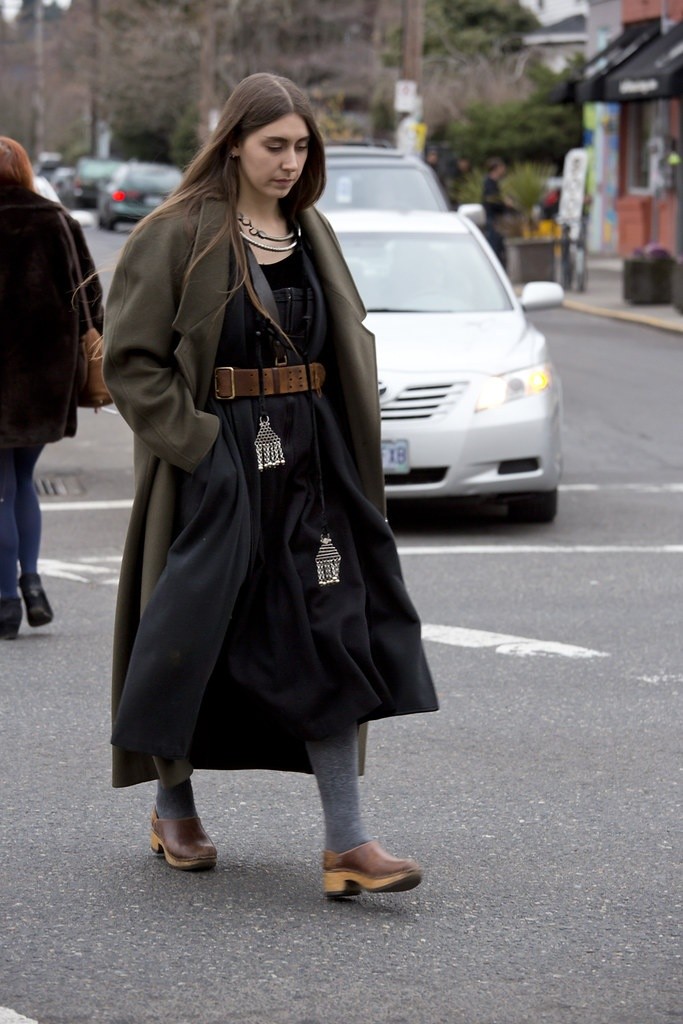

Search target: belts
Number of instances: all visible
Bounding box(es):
[211,363,327,401]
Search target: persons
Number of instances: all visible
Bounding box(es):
[0,137,102,641]
[101,73,440,898]
[482,158,507,250]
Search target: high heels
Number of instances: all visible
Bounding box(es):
[322,841,422,897]
[150,806,217,869]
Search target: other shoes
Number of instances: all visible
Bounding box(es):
[18,572,53,626]
[0,598,23,639]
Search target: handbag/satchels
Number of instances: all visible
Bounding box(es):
[75,328,113,407]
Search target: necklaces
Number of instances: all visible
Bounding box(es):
[239,212,298,251]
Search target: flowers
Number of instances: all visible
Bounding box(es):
[621,246,675,262]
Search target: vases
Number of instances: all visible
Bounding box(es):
[623,261,673,305]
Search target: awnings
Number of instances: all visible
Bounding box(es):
[545,24,642,105]
[604,20,683,99]
[575,24,661,102]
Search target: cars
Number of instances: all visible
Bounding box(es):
[312,143,450,215]
[37,152,125,211]
[316,209,564,527]
[96,163,187,231]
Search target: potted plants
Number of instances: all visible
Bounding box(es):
[499,160,563,284]
